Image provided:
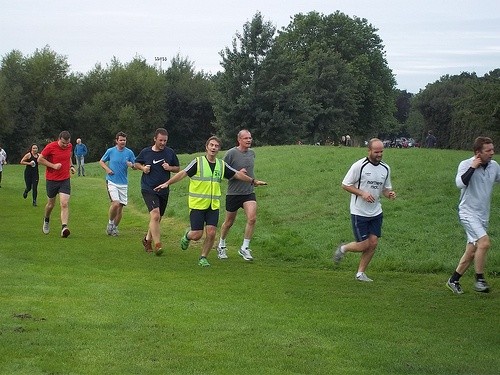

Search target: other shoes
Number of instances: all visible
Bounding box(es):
[23,190,28,199]
[78,174,80,176]
[33,201,36,206]
[82,174,85,176]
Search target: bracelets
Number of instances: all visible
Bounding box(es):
[71,166,74,168]
[251,179,255,185]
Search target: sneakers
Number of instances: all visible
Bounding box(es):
[181,230,189,250]
[198,257,210,267]
[142,236,154,252]
[61,226,70,238]
[106,223,114,235]
[43,218,50,234]
[334,243,346,262]
[217,246,228,259]
[238,246,253,260]
[474,278,490,293]
[355,272,373,282]
[154,243,163,256]
[446,278,465,295]
[111,226,119,236]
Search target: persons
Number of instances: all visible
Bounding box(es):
[217,128,256,260]
[384,140,420,148]
[445,136,500,294]
[342,134,351,146]
[134,128,180,255]
[99,132,136,236]
[74,138,87,176]
[334,138,396,281]
[20,143,41,206]
[37,131,76,238]
[153,136,268,266]
[0,147,7,188]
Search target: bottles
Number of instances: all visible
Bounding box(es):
[31,160,35,167]
